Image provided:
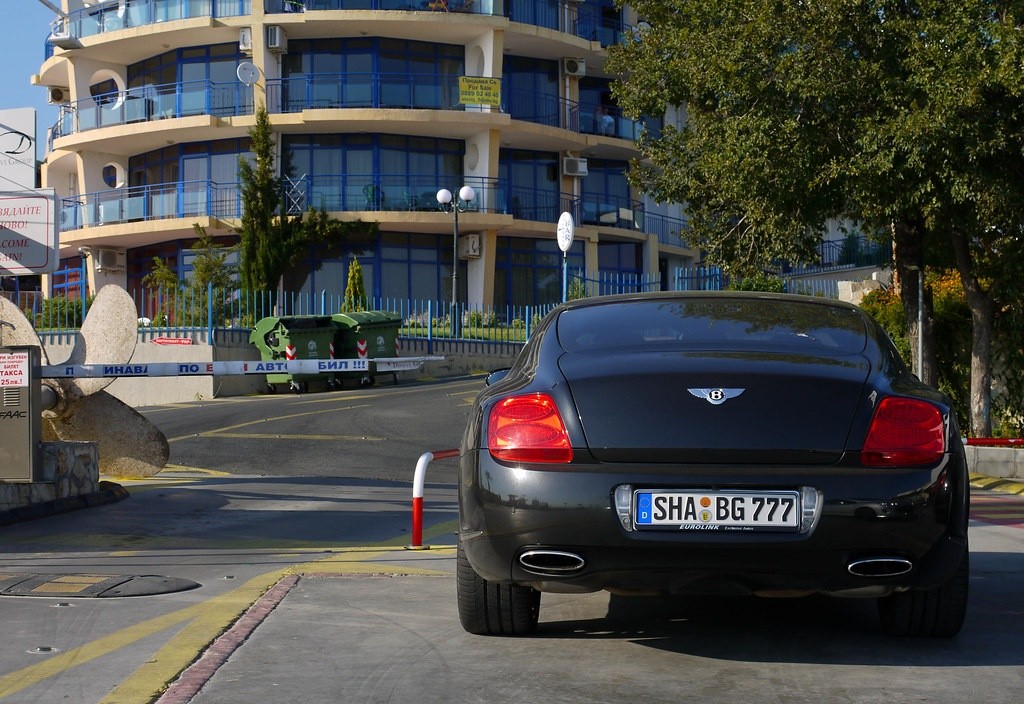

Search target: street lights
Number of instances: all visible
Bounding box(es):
[434,185,475,341]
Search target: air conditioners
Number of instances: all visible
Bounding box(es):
[268,25,288,53]
[561,157,588,176]
[560,57,586,77]
[239,27,251,50]
[47,86,70,103]
[94,248,126,272]
[457,234,480,259]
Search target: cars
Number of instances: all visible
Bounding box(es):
[451,288,973,642]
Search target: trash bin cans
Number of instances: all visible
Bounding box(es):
[334,311,405,387]
[248,313,341,394]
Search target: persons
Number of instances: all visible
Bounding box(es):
[593,106,614,134]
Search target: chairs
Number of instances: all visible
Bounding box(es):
[362,185,386,211]
[403,191,420,211]
